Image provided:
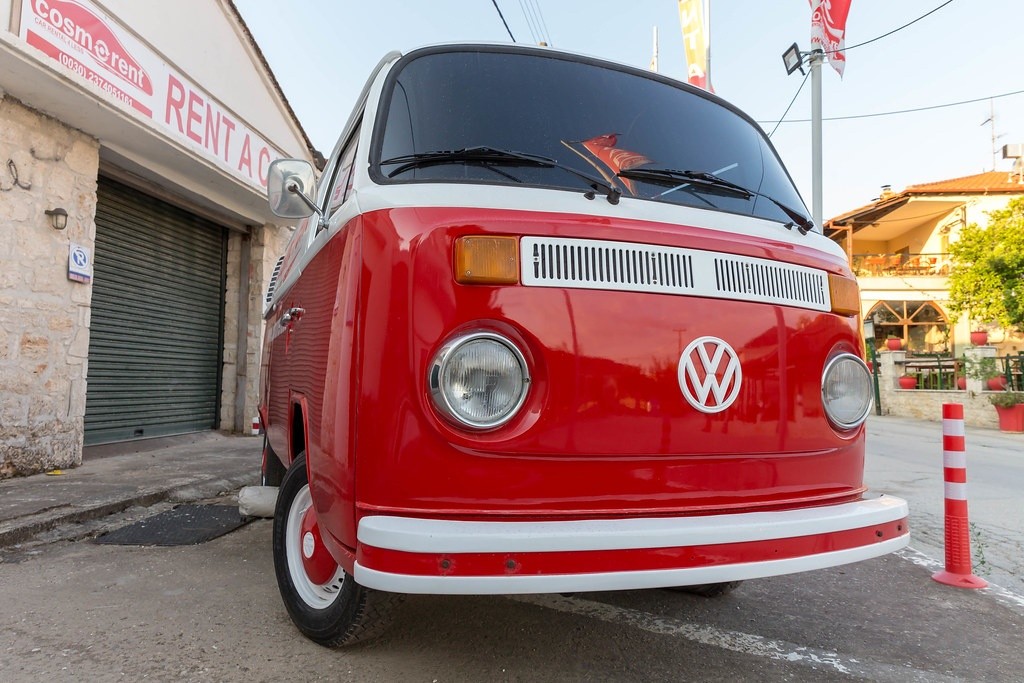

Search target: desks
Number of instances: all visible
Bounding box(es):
[897,266,933,275]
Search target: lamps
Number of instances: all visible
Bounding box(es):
[44,208,66,230]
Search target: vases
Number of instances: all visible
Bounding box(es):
[912,258,919,264]
[929,258,936,263]
[971,332,987,345]
[989,393,1024,431]
[887,338,901,350]
[890,256,900,264]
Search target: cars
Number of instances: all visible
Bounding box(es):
[259,42,910,647]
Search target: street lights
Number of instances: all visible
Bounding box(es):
[778,44,829,233]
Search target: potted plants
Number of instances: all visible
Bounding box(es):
[955,356,973,389]
[866,343,874,374]
[900,372,918,389]
[973,357,1007,390]
